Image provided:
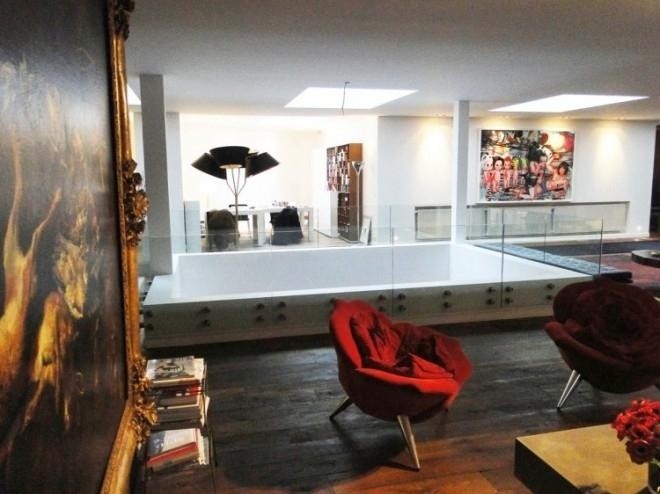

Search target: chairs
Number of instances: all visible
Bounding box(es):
[329,298,473,471]
[270,205,303,245]
[207,210,240,250]
[543,277,660,411]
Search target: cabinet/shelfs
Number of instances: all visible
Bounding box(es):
[140,357,218,493]
[327,142,363,242]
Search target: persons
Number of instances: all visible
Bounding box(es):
[504,152,570,200]
[525,148,549,199]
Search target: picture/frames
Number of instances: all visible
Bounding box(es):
[0,0,160,494]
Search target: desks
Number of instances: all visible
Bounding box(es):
[205,204,314,247]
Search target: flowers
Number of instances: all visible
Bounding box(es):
[612,397,660,465]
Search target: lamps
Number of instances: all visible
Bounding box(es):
[191,144,280,248]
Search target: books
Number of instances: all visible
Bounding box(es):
[136,351,212,483]
